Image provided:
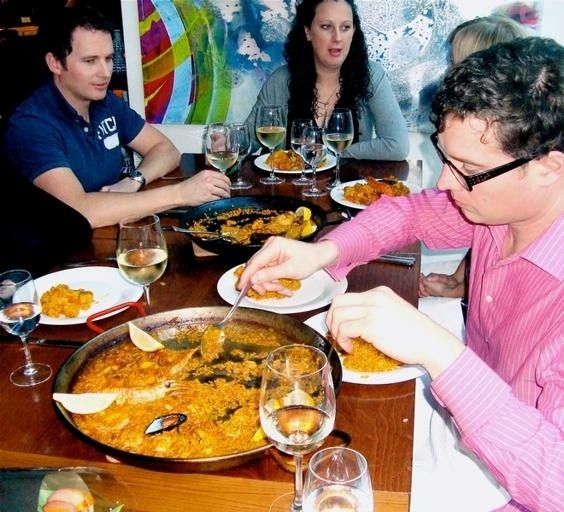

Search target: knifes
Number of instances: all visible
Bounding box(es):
[0,335,85,348]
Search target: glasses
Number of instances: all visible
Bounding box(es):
[431,130,543,191]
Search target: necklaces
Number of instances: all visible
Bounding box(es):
[317,84,339,114]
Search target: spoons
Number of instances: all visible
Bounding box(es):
[200,265,266,361]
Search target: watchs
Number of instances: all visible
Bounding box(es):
[128,170,145,191]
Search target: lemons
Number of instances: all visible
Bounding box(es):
[300,222,315,237]
[126,321,164,352]
[295,207,313,221]
[250,389,318,443]
[53,392,116,414]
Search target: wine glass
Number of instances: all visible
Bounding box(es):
[0,269,54,387]
[255,104,287,186]
[228,123,253,192]
[116,213,169,317]
[322,109,355,190]
[299,126,329,197]
[205,122,240,175]
[291,121,314,186]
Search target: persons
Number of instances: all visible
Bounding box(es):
[414,12,534,299]
[7,5,233,231]
[232,36,564,511]
[208,1,413,163]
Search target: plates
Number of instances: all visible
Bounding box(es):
[11,265,147,326]
[329,178,424,211]
[252,146,340,174]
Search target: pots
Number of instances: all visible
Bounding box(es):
[50,299,352,474]
[177,193,353,261]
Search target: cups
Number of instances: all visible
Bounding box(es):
[302,446,374,512]
[258,343,337,512]
